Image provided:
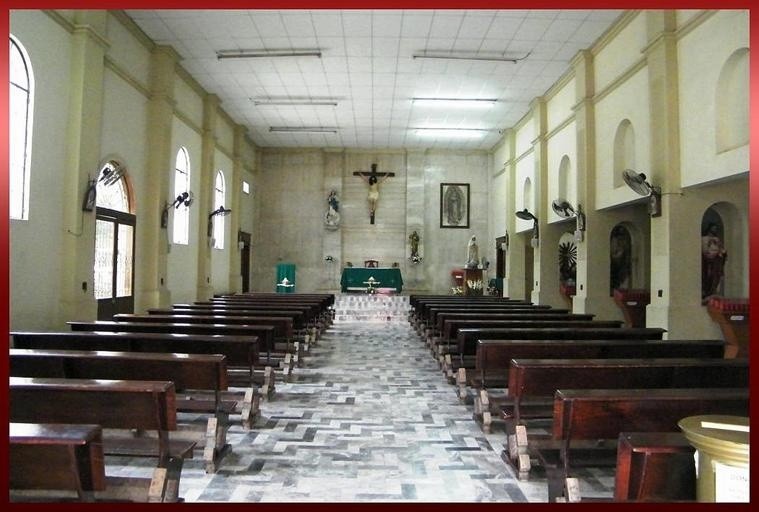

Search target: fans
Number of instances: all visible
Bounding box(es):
[621,169,661,218]
[516,208,538,239]
[82,164,127,212]
[551,198,585,231]
[162,192,192,228]
[209,204,232,233]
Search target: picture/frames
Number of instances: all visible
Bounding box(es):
[439,182,470,229]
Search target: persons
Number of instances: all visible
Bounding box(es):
[358,170,391,216]
[445,186,462,224]
[468,235,480,268]
[409,230,420,257]
[699,223,728,305]
[325,189,341,223]
[608,224,635,292]
